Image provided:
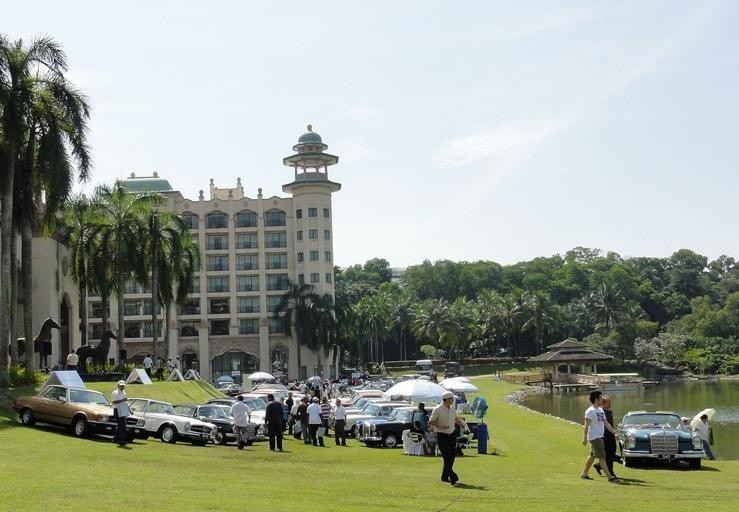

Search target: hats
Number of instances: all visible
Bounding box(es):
[118,380,125,386]
[313,397,319,401]
[443,392,458,399]
[682,417,690,422]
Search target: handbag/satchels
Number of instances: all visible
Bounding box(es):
[117,403,130,417]
[709,429,713,445]
[318,428,325,436]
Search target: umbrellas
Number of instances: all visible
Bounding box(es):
[381,379,459,400]
[690,409,715,432]
[440,377,478,392]
[248,372,275,383]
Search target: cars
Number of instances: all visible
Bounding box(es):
[616,408,703,470]
[172,357,466,451]
[122,397,218,446]
[14,384,125,440]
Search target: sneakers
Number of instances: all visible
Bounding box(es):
[608,477,624,483]
[594,464,602,475]
[580,475,593,480]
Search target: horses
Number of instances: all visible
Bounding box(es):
[9,317,61,369]
[75,330,116,373]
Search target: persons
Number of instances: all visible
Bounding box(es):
[694,414,715,460]
[593,395,616,476]
[66,349,79,370]
[111,380,133,445]
[412,403,470,457]
[429,390,462,485]
[143,354,181,381]
[676,416,691,430]
[230,396,252,449]
[264,380,347,451]
[581,391,619,482]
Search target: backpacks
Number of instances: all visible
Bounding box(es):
[413,413,426,430]
